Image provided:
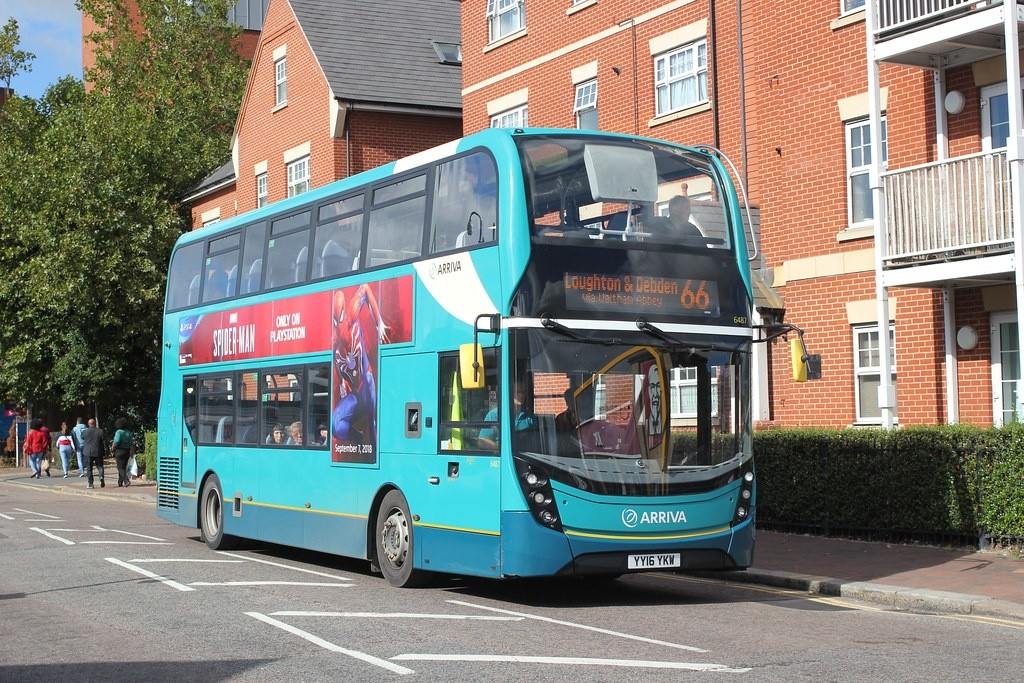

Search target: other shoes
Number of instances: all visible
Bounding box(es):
[45,470,50,476]
[79,474,84,477]
[86,485,94,489]
[36,476,40,478]
[63,475,67,478]
[119,483,123,486]
[124,481,131,487]
[30,471,38,478]
[100,476,105,487]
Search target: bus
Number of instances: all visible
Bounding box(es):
[156,127,824,601]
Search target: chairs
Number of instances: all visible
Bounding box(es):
[642,215,668,244]
[193,423,329,448]
[352,249,422,270]
[455,228,477,251]
[188,238,349,304]
[464,408,489,451]
[604,213,637,242]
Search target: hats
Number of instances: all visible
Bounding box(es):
[316,421,327,430]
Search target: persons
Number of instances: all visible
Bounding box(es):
[477,380,533,450]
[54,417,109,488]
[112,417,135,487]
[23,419,52,478]
[332,283,391,463]
[668,195,703,235]
[555,387,585,459]
[272,421,328,446]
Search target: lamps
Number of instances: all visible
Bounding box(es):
[944,90,966,115]
[957,326,979,351]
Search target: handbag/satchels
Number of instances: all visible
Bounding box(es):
[50,455,55,463]
[41,457,49,470]
[126,454,138,477]
